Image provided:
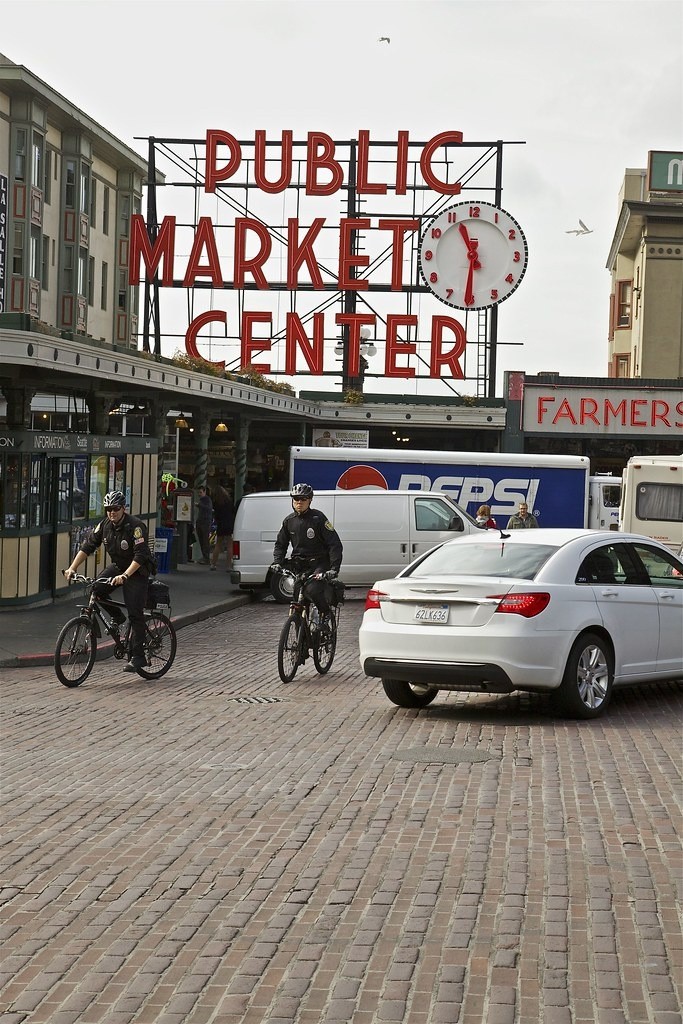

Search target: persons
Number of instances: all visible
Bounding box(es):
[475,505,497,529]
[193,485,212,565]
[64,491,152,672]
[210,486,235,572]
[271,483,343,659]
[506,503,539,529]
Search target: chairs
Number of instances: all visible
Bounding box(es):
[576,548,619,582]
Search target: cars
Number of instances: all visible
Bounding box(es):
[359,528,683,718]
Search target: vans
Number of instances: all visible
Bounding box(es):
[229,489,500,604]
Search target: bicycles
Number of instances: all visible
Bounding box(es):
[54,569,177,688]
[267,567,340,683]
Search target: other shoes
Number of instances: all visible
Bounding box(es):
[104,611,127,635]
[197,558,210,564]
[316,609,332,631]
[122,655,147,672]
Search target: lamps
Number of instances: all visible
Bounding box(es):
[215,408,229,432]
[632,287,641,292]
[173,407,188,428]
[127,397,148,417]
[141,400,151,415]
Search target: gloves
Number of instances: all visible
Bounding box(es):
[271,564,284,575]
[326,569,337,580]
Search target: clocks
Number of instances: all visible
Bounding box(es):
[417,200,528,312]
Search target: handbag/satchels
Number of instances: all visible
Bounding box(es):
[146,580,170,609]
[327,580,347,608]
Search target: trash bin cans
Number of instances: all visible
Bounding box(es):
[155,527,174,574]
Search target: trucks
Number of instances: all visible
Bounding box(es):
[289,446,622,532]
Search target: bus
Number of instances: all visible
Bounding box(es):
[618,454,683,582]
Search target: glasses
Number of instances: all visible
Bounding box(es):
[293,496,309,501]
[106,506,121,512]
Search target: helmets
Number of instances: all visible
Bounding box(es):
[290,483,314,499]
[102,490,126,507]
